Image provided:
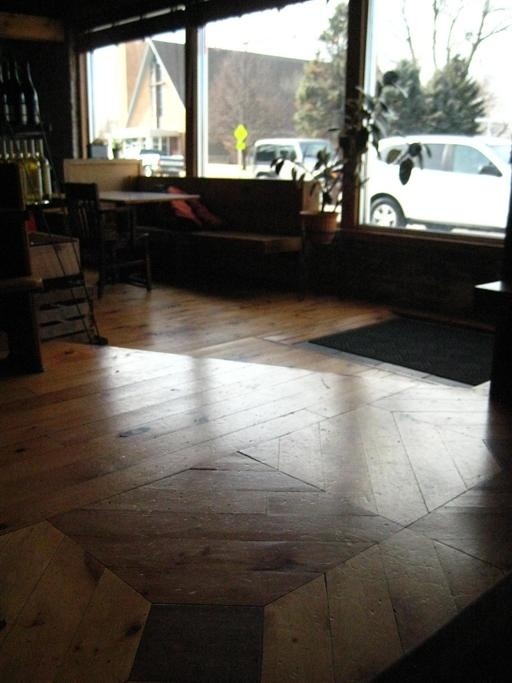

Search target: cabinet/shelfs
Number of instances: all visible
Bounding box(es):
[0,123,69,215]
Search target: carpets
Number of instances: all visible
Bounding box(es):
[307,315,497,386]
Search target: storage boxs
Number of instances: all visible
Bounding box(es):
[27,230,81,280]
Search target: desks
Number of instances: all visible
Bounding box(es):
[52,190,201,284]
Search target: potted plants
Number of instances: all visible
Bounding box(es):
[270,71,433,246]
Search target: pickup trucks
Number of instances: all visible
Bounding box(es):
[118,148,184,177]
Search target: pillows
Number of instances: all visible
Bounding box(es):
[166,184,202,230]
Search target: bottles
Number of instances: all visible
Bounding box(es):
[0,58,54,205]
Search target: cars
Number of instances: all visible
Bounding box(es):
[250,135,511,239]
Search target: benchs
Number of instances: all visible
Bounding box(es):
[130,174,320,289]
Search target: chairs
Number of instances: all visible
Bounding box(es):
[62,181,152,300]
